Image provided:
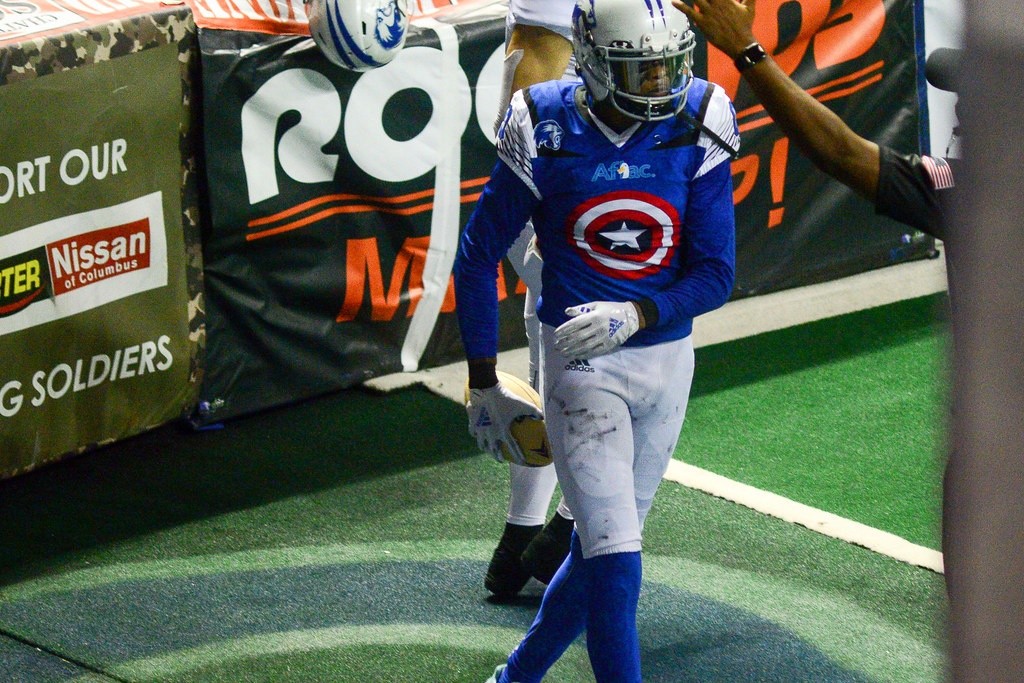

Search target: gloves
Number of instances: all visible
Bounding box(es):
[552,301,640,362]
[466,382,546,466]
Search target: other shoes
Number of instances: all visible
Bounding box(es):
[484,663,519,683]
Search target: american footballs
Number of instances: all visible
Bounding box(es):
[463,370,554,468]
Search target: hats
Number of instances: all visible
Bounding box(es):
[926,45,981,95]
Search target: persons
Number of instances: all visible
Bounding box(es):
[305,0,742,683]
[667,0,974,601]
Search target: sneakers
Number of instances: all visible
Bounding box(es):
[485,537,533,598]
[520,521,572,587]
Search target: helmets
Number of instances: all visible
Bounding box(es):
[571,0,697,120]
[304,0,415,73]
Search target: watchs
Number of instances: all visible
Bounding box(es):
[733,41,768,73]
